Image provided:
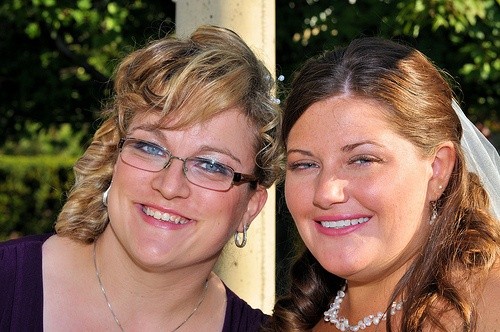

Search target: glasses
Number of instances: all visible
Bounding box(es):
[119,138,258,192]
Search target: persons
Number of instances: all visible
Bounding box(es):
[261,38,500,332]
[1,24,289,332]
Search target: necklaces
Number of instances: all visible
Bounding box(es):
[93,236,207,332]
[322,280,410,332]
